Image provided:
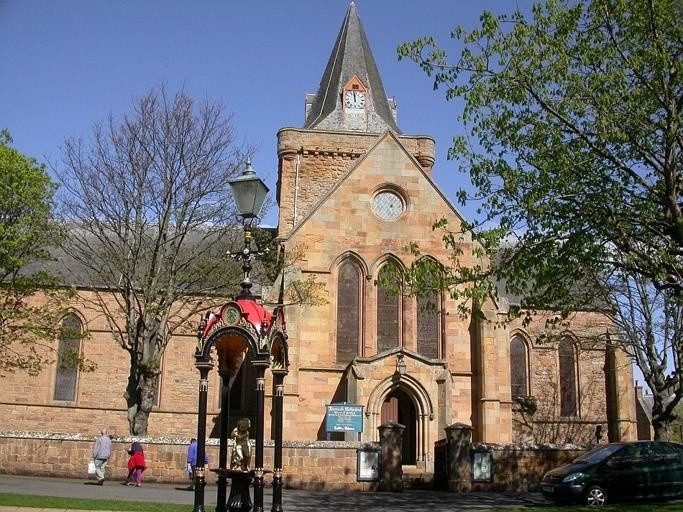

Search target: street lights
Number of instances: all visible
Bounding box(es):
[225,157,271,297]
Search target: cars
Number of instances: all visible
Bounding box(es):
[539,440,683,506]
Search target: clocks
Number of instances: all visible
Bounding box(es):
[342,75,368,111]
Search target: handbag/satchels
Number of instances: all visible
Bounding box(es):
[88,461,96,474]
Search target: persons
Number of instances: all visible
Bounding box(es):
[232,417,253,474]
[91,429,121,485]
[121,437,148,487]
[187,439,208,490]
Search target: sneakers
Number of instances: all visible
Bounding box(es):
[137,484,141,486]
[98,478,105,485]
[187,487,193,490]
[126,483,130,485]
